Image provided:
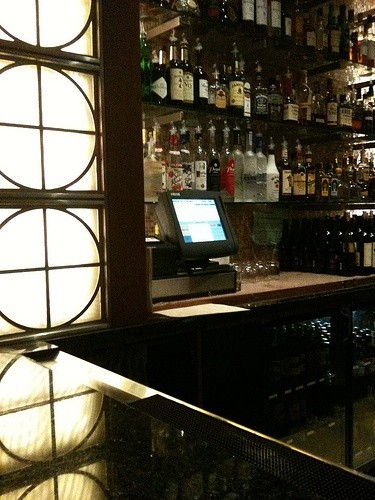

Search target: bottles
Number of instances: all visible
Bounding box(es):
[138,0,375,202]
[281,210,375,276]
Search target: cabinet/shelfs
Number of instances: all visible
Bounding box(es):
[139,4,375,207]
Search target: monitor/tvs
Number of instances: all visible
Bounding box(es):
[153,190,238,261]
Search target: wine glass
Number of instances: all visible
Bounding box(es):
[232,246,279,282]
